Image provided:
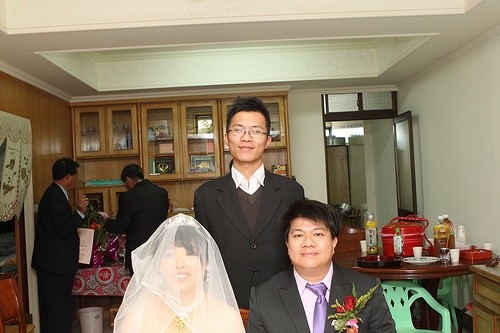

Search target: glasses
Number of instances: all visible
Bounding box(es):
[226,129,267,136]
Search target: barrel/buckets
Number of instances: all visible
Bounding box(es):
[377,217,430,257]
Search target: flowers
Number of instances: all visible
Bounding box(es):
[328,283,378,333]
[73,203,114,244]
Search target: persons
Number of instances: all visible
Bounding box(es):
[193,96,304,310]
[246,199,397,333]
[30,157,89,333]
[103,164,169,278]
[112,213,246,333]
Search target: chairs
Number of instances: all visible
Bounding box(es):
[0,272,36,333]
[380,277,459,333]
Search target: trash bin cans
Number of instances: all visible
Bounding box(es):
[78,307,104,333]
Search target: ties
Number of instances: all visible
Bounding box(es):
[306,282,329,333]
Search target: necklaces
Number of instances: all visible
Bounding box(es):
[165,305,196,330]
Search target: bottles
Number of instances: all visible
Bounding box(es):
[444,214,455,249]
[362,210,377,246]
[433,215,449,256]
[393,228,403,257]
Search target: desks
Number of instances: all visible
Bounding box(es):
[332,249,497,330]
[71,261,132,296]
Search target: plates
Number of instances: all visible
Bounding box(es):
[461,248,492,259]
[355,255,402,268]
[402,256,440,266]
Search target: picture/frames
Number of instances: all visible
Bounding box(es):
[194,111,214,134]
[189,154,215,172]
[151,157,175,174]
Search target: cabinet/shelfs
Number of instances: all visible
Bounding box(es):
[70,90,292,219]
[469,264,500,333]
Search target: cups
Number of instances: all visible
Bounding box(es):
[360,240,367,252]
[440,247,450,266]
[456,226,466,249]
[449,249,460,263]
[483,243,493,251]
[412,246,423,257]
